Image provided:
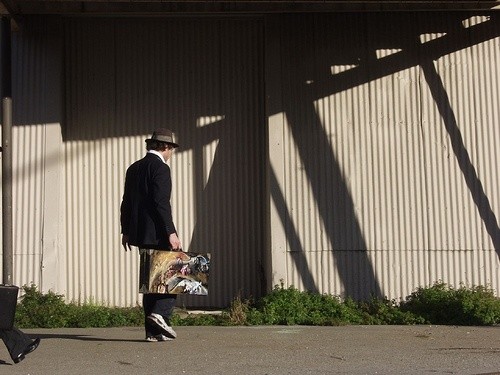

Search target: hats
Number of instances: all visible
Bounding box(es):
[145,127,179,147]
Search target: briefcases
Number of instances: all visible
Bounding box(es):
[0,283,19,331]
[139,247,211,293]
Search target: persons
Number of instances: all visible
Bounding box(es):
[120,126,183,343]
[0,325,41,365]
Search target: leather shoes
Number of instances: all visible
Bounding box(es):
[13,338,40,364]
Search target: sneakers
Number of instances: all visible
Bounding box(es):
[145,333,173,342]
[147,313,176,339]
